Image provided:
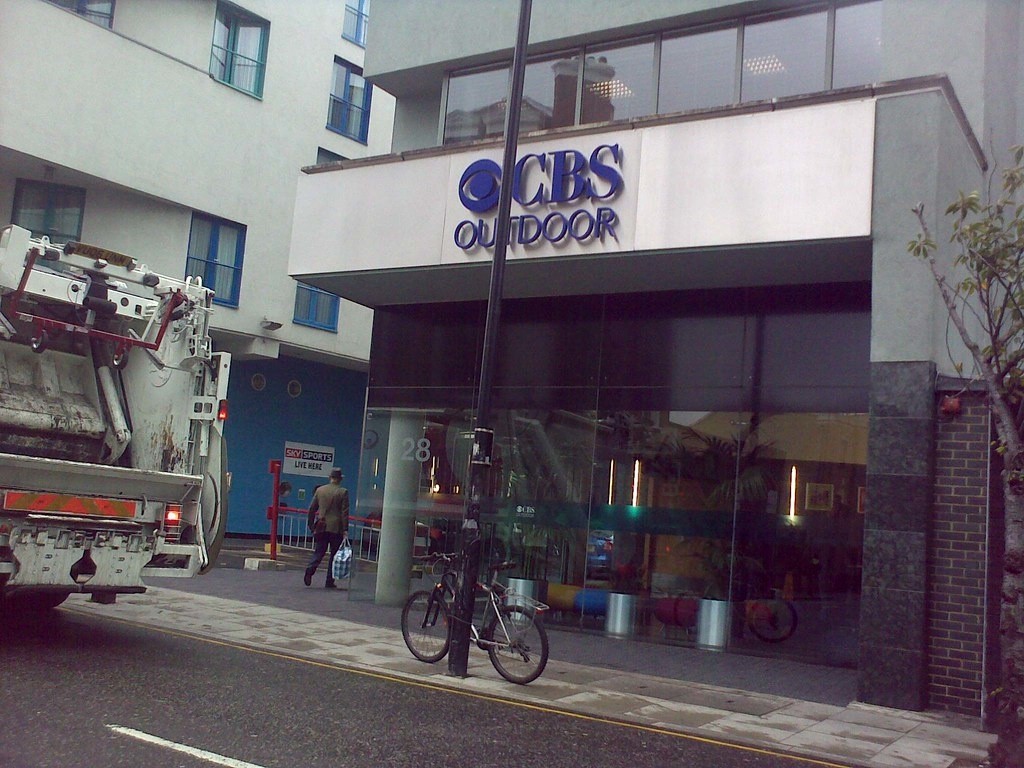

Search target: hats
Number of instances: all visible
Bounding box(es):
[328,468,345,477]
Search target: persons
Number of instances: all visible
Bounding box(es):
[304,467,350,589]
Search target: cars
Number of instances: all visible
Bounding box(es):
[585,528,618,578]
[362,509,459,557]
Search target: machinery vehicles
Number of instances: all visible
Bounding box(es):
[0,220,234,615]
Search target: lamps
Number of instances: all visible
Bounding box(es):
[261,321,283,332]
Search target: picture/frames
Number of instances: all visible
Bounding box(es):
[857,486,865,514]
[804,483,835,512]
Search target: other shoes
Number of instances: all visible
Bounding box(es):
[325,584,337,588]
[304,567,312,586]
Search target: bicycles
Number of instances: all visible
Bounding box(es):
[686,546,798,642]
[400,545,552,687]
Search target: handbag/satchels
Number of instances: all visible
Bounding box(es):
[311,517,326,542]
[333,537,353,581]
